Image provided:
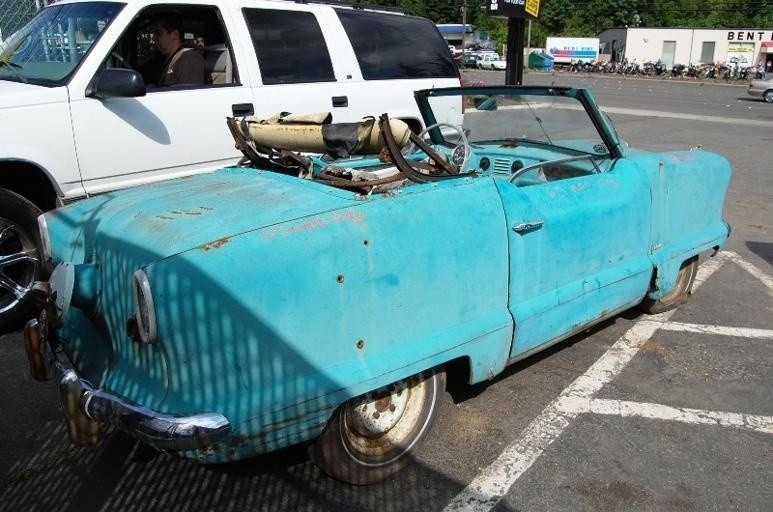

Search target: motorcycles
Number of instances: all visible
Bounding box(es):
[568,58,762,81]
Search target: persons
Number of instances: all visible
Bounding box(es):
[147,16,206,86]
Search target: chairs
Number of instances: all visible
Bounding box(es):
[202,24,232,84]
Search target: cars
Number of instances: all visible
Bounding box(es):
[22,85,733,486]
[448,44,507,70]
[747,74,773,102]
